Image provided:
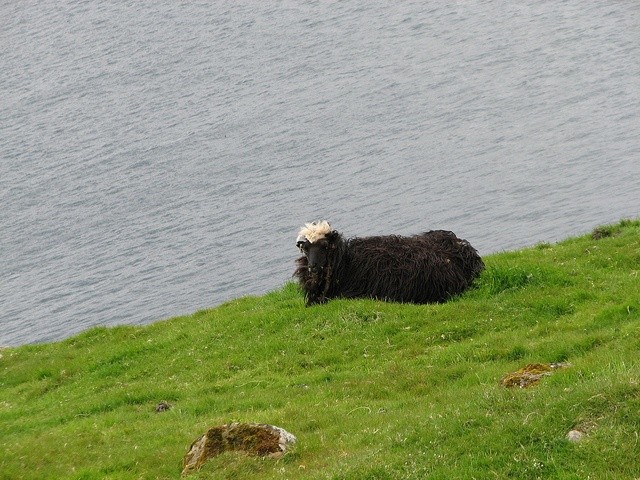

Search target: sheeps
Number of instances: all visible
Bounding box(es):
[290,218,488,309]
